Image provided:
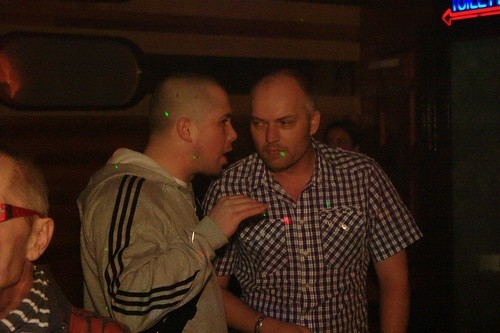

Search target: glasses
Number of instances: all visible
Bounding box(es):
[0,203,39,223]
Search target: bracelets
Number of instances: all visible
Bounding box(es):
[254,315,267,333]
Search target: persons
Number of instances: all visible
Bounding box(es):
[323,119,364,156]
[200,70,424,333]
[75,72,270,333]
[0,146,131,333]
